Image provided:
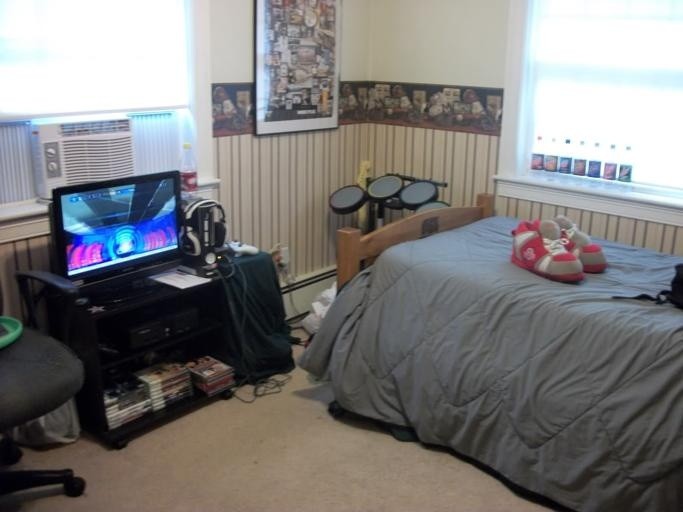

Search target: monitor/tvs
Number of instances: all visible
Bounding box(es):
[53,170,185,306]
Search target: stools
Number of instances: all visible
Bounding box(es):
[0,325,86,508]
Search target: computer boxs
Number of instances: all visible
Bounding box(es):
[177,195,221,276]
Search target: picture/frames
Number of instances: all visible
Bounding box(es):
[253,2,340,137]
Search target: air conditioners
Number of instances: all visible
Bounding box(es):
[28,113,134,207]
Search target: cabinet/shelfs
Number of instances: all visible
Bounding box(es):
[16,248,275,449]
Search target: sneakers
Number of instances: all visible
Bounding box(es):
[532,215,607,273]
[511,222,584,281]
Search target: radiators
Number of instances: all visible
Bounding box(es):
[0,109,184,220]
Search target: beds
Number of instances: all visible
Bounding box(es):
[335,193,683,512]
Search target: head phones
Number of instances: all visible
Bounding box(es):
[176,198,227,256]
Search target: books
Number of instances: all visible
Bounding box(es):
[102,354,236,430]
